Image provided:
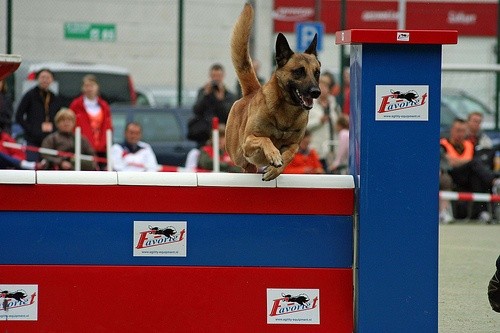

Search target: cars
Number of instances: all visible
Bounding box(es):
[136,90,200,108]
[109,103,200,166]
[441,87,500,172]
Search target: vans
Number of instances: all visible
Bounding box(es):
[23,60,136,110]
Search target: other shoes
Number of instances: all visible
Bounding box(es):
[439,203,456,223]
[481,210,494,224]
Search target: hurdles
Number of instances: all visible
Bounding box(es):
[1,28,459,333]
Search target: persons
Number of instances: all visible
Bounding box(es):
[186,66,244,173]
[70,74,113,172]
[15,69,63,164]
[0,75,46,170]
[297,65,351,175]
[110,121,159,172]
[40,109,98,171]
[439,113,500,224]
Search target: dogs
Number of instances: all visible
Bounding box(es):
[226,3,321,181]
